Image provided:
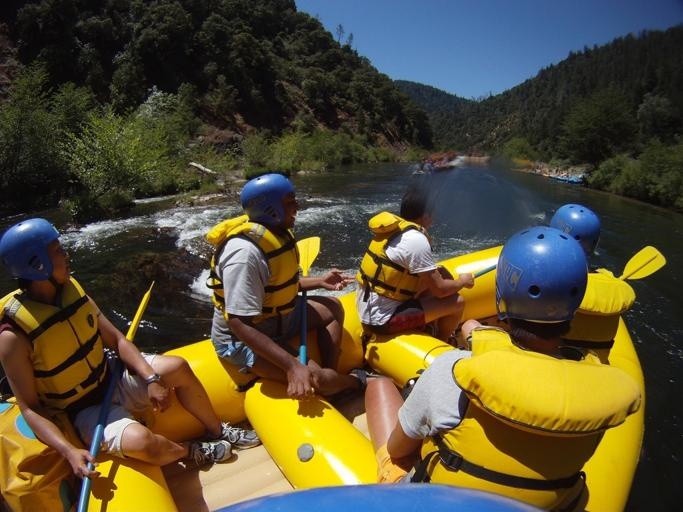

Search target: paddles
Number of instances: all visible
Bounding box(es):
[292,235,316,402]
[615,246,666,282]
[76,280,155,512]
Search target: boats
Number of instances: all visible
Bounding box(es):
[548,170,590,185]
[413,155,464,173]
[0,243,647,512]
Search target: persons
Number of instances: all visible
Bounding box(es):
[0,218,261,481]
[365,226,641,512]
[206,174,381,400]
[356,186,474,349]
[531,160,569,178]
[461,204,636,365]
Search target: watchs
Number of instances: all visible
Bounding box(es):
[145,373,161,384]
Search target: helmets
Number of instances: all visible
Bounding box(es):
[240,174,295,227]
[549,203,603,256]
[495,225,588,325]
[0,219,61,281]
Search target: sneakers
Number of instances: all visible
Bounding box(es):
[206,422,261,450]
[348,368,384,393]
[184,440,233,463]
